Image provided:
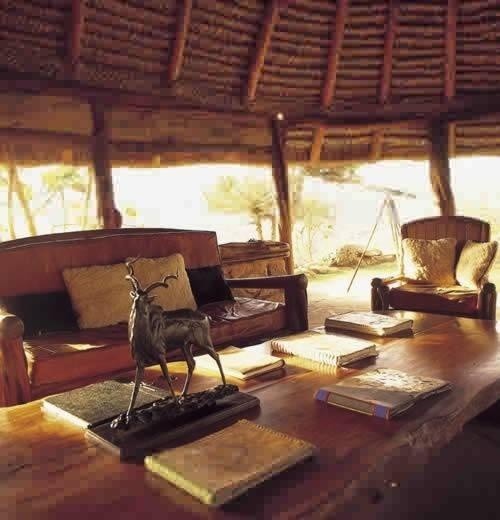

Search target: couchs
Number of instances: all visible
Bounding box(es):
[1,228,310,407]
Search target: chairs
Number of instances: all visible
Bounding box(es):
[369,217,497,319]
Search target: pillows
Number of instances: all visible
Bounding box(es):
[401,238,457,283]
[61,263,137,329]
[455,239,498,291]
[128,253,198,314]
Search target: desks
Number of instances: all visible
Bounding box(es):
[0,306,500,520]
[218,240,292,278]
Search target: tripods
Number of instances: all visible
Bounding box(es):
[347,195,401,293]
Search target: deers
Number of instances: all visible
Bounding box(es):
[110,254,228,427]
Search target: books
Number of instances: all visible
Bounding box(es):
[270,333,378,365]
[224,368,285,384]
[194,345,285,380]
[272,350,376,377]
[41,379,171,430]
[143,418,317,509]
[315,367,452,421]
[324,310,413,336]
